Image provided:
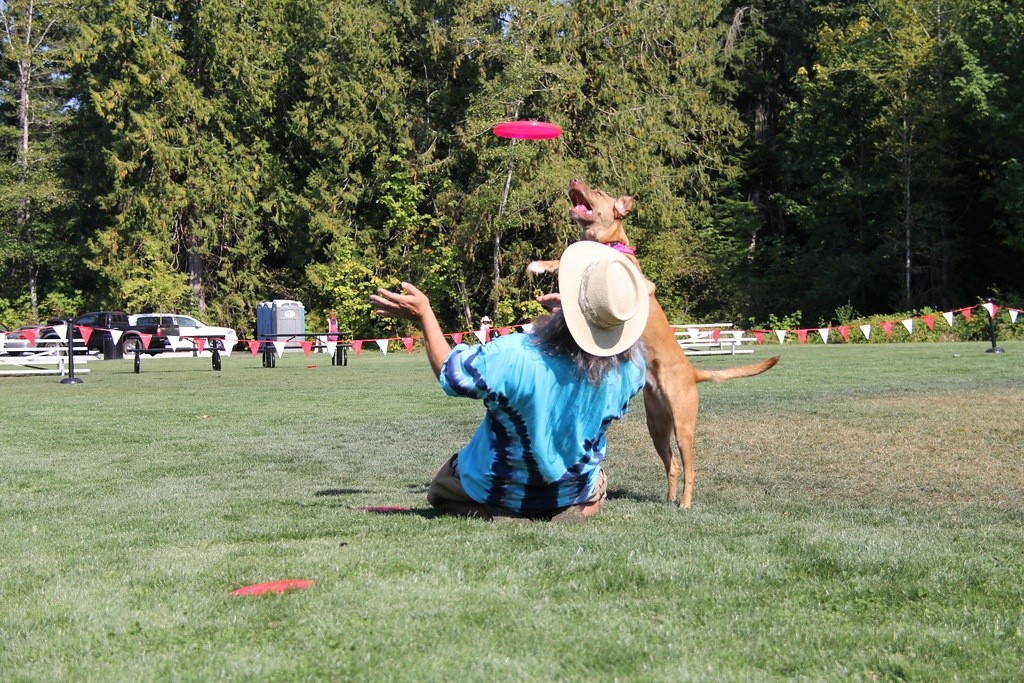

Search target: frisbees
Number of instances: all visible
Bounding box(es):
[493,121,563,140]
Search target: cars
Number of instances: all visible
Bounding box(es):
[1,326,43,355]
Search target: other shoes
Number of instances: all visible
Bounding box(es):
[550,506,586,523]
[490,509,526,525]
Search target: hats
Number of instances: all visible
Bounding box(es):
[558,240,649,357]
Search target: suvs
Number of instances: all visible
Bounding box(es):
[127,314,238,353]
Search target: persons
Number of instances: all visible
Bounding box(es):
[370,240,650,524]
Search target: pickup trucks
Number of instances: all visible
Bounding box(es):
[39,310,184,355]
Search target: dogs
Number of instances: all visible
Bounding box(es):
[566,177,781,510]
